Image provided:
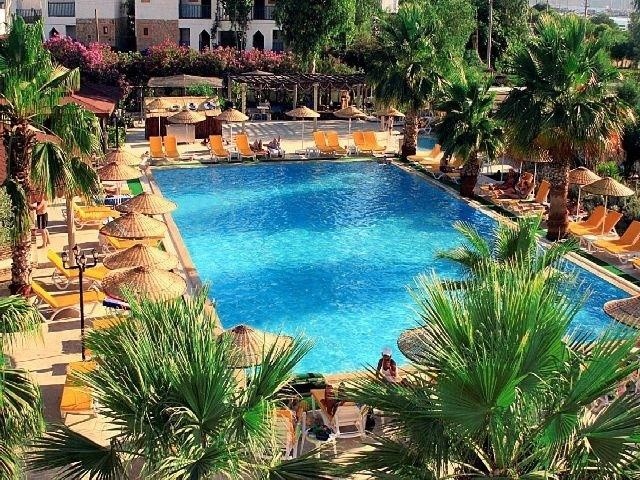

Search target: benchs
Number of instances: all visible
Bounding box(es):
[60,361,98,421]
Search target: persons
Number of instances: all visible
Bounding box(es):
[376,348,397,382]
[27,199,50,249]
[387,117,393,135]
[489,173,530,198]
[440,153,464,173]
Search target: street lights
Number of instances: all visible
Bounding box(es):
[114,108,125,148]
[61,243,99,360]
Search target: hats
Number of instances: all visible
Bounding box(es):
[382,348,392,356]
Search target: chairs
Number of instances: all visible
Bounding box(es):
[352,130,387,155]
[148,135,179,162]
[477,169,552,226]
[312,130,348,157]
[255,387,373,457]
[209,134,257,164]
[567,204,640,277]
[406,143,469,185]
[28,193,122,332]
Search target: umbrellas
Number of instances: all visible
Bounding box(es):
[397,327,435,367]
[569,166,634,235]
[333,106,367,148]
[216,324,293,369]
[215,108,249,147]
[167,108,207,155]
[95,148,186,305]
[604,295,640,330]
[285,108,320,150]
[372,108,405,139]
